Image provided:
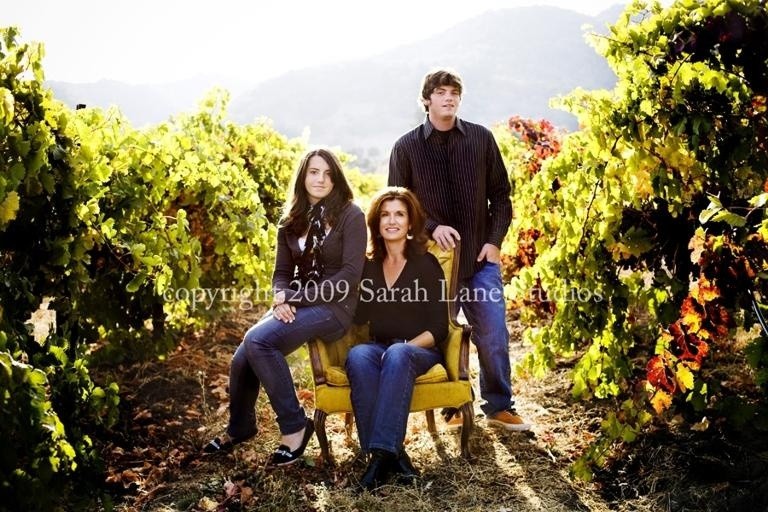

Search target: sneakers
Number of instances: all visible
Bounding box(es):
[359,451,393,488]
[393,451,417,483]
[449,418,464,427]
[204,427,258,453]
[273,418,314,466]
[485,410,530,430]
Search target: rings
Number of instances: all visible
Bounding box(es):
[275,309,279,312]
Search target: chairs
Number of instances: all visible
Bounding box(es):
[303,235,479,471]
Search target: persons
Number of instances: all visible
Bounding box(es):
[197,147,368,472]
[385,71,537,434]
[342,184,451,492]
